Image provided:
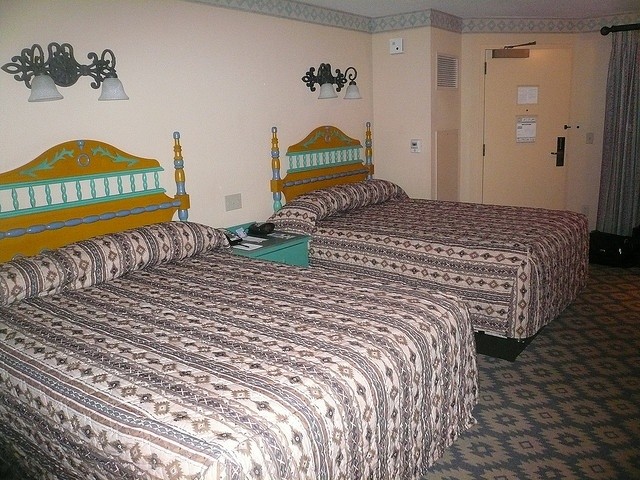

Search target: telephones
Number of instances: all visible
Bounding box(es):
[218,228,242,245]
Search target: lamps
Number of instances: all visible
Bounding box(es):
[97,77,130,102]
[342,81,363,100]
[24,74,64,102]
[317,82,339,100]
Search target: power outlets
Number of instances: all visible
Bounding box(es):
[224,193,242,212]
[587,132,594,144]
[410,138,423,154]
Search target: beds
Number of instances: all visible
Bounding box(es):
[0,132,478,477]
[270,123,589,362]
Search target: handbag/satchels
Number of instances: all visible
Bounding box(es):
[590,230,638,268]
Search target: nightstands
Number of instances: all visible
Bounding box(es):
[218,222,313,269]
